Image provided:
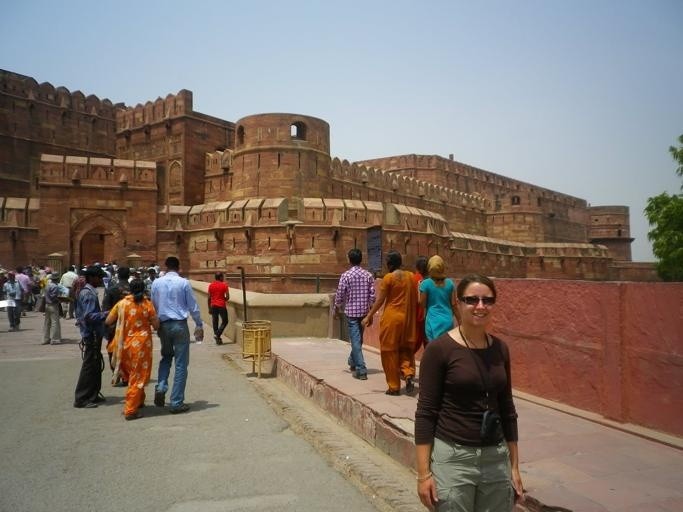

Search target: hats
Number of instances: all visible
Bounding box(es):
[87,265,107,279]
[49,272,60,280]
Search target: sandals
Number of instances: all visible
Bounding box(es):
[126,411,144,420]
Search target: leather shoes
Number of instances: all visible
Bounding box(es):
[90,395,105,404]
[85,402,98,408]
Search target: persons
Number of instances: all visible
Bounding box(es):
[413,274,525,512]
[400,254,430,380]
[329,248,377,381]
[361,249,419,395]
[417,255,462,344]
[207,271,230,345]
[0,256,205,422]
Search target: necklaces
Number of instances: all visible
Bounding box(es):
[465,333,487,350]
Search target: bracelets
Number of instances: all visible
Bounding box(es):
[415,471,433,483]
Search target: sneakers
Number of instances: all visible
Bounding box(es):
[386,389,400,396]
[352,372,367,380]
[154,390,165,406]
[348,357,356,371]
[405,375,414,393]
[214,334,221,345]
[169,403,190,414]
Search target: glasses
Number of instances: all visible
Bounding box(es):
[460,296,495,305]
